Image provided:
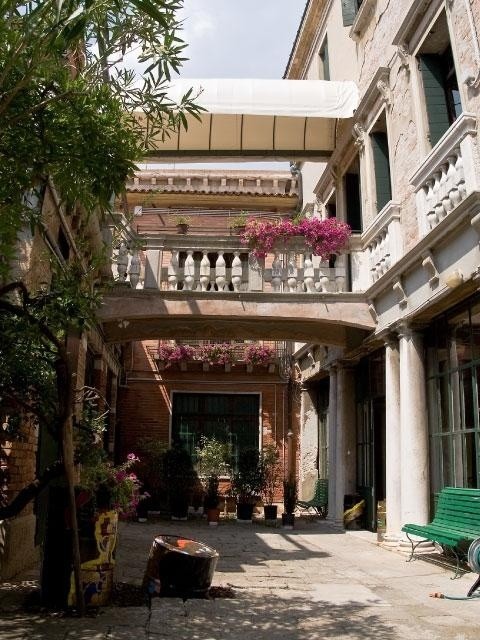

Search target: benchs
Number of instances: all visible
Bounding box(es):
[402,486,480,581]
[295,478,329,521]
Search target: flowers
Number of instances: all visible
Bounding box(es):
[239,216,352,264]
[156,342,277,370]
[82,450,151,521]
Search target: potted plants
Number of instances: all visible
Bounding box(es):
[167,213,193,235]
[136,433,299,530]
[226,210,248,235]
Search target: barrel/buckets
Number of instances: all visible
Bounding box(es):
[141,533,221,601]
[41,492,118,613]
